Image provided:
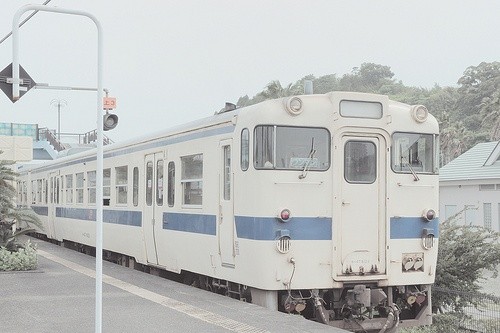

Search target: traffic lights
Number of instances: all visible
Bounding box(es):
[103,113,118,131]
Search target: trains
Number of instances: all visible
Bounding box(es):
[5,80,441,333]
